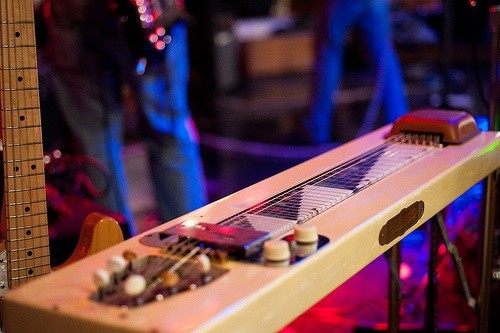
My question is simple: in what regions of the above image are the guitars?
[0,1,126,295]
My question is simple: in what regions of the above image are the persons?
[303,0,411,146]
[48,0,204,235]
